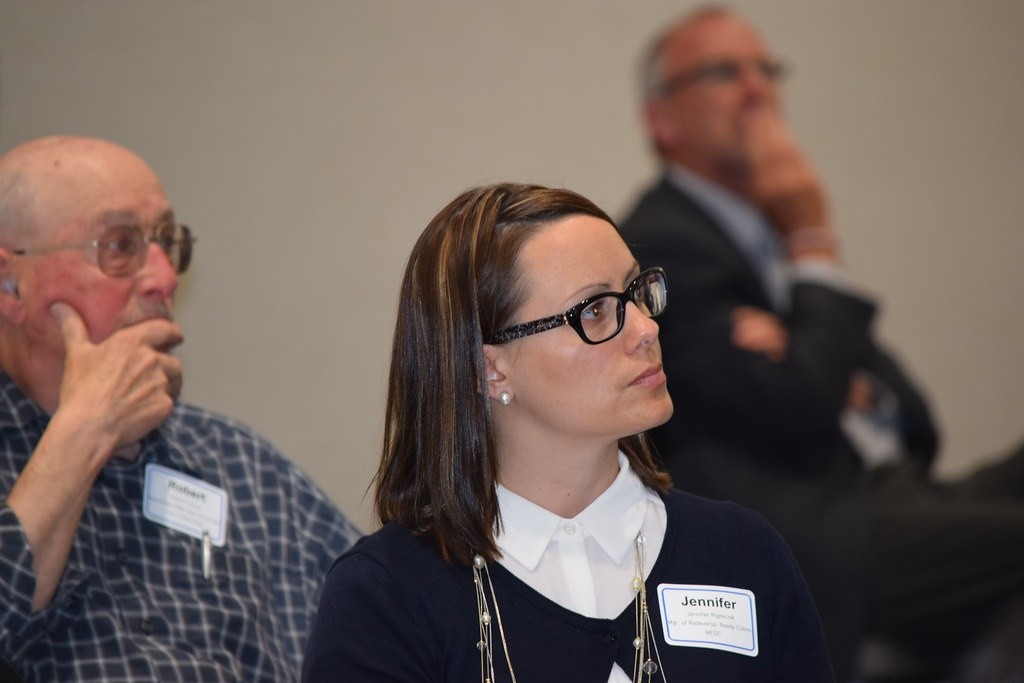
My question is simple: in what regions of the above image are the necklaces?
[472,533,666,683]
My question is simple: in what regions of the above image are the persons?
[616,12,1023,683]
[300,182,835,683]
[0,135,363,683]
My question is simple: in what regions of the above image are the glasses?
[484,266,668,345]
[6,222,197,277]
[659,56,788,97]
